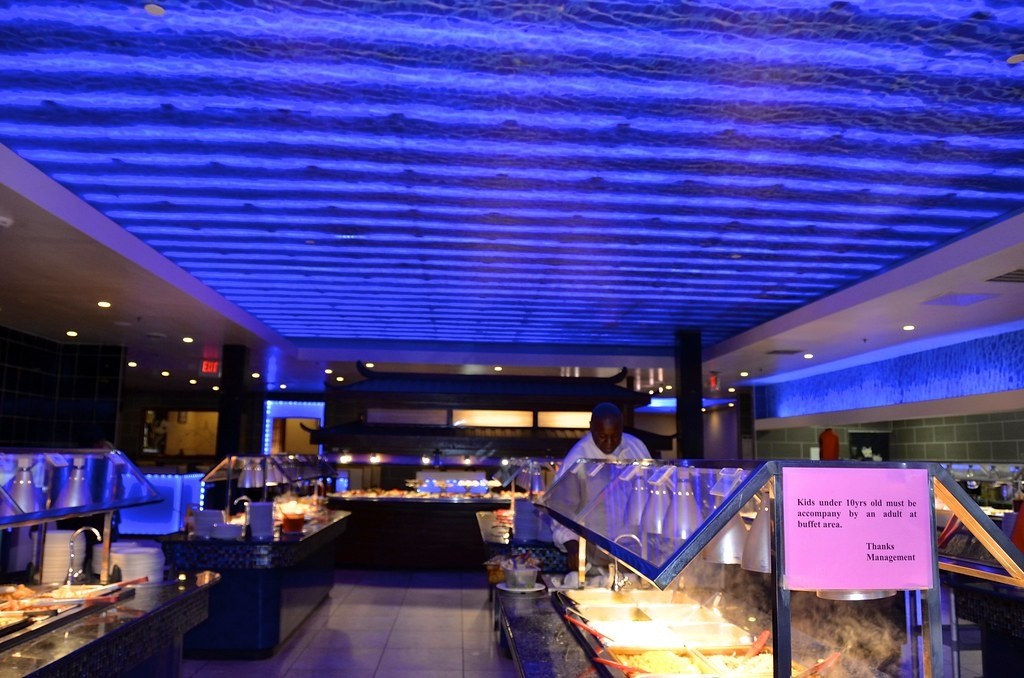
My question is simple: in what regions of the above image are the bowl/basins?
[503,567,538,588]
[283,512,306,533]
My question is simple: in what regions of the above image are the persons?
[547,402,671,585]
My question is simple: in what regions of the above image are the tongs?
[31,596,120,607]
[77,575,148,595]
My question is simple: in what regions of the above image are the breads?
[0,584,34,601]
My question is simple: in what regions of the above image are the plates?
[496,582,547,592]
[194,510,242,540]
[30,529,165,584]
[249,501,275,540]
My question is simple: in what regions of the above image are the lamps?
[51,458,92,509]
[0,458,35,515]
[517,466,546,492]
[967,464,1024,489]
[623,470,898,601]
[238,462,323,488]
